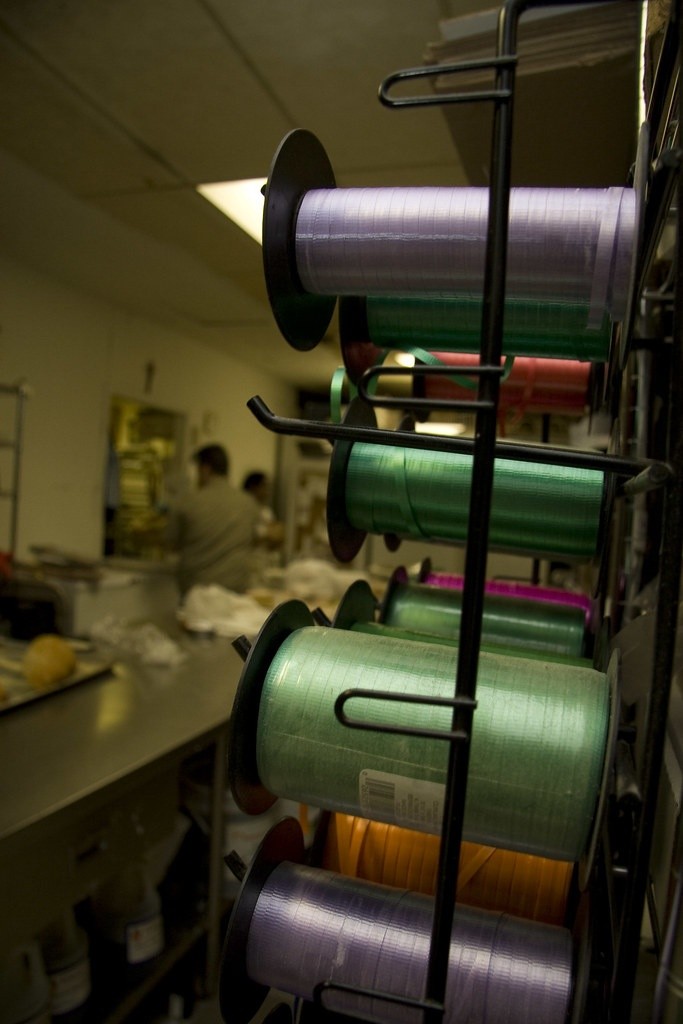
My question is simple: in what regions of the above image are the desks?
[0,611,298,1024]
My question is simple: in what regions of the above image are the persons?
[163,445,276,642]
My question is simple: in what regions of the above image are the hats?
[195,446,227,474]
